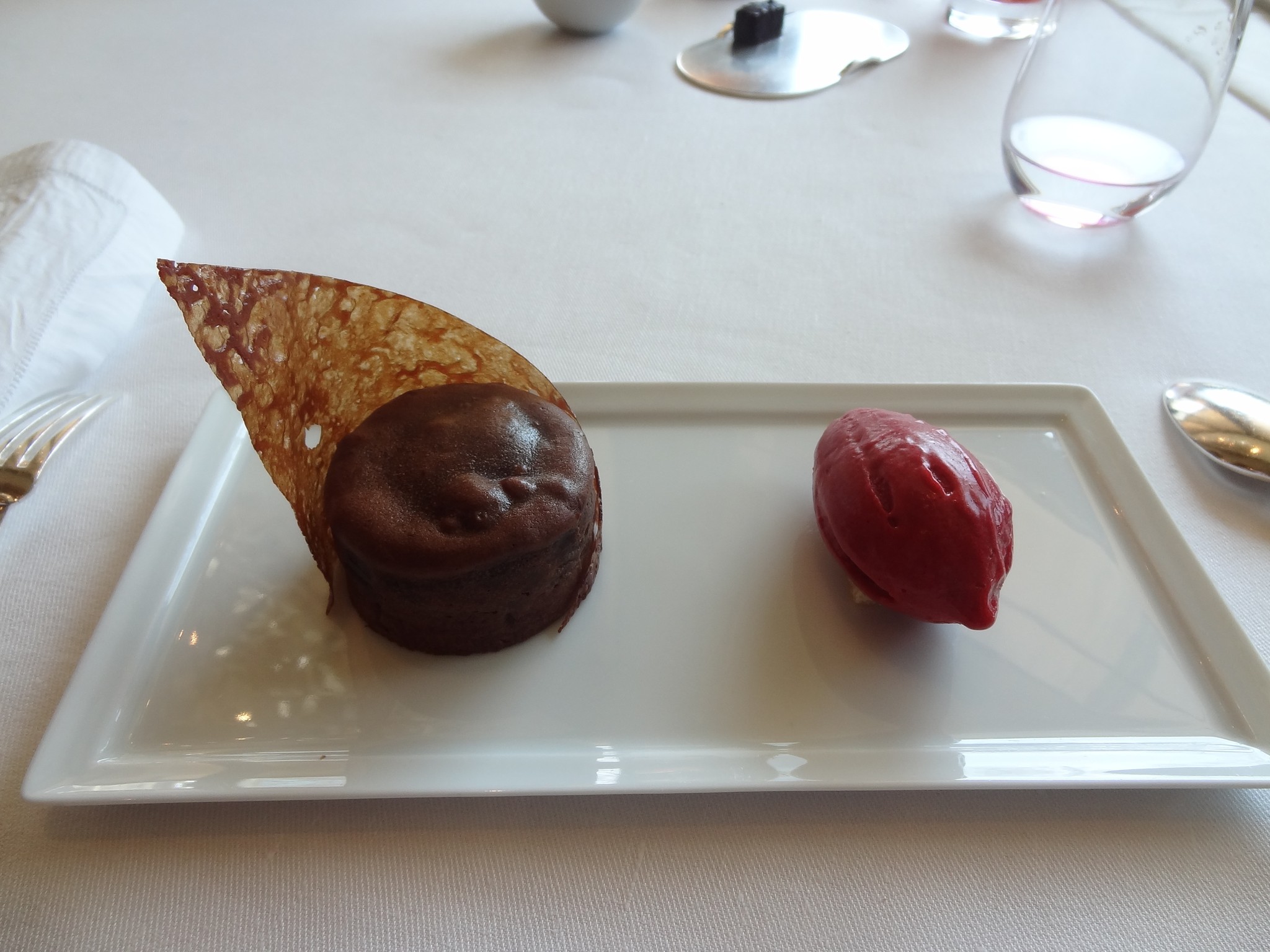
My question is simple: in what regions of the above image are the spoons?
[1163,379,1270,481]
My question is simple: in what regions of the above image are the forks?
[0,387,107,522]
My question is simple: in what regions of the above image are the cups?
[1003,0,1253,229]
[948,0,1062,40]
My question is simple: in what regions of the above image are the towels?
[0,134,187,429]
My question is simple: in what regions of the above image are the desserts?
[155,258,605,658]
[813,406,1013,630]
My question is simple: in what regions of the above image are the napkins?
[0,140,185,417]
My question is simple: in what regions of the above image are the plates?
[20,380,1270,808]
